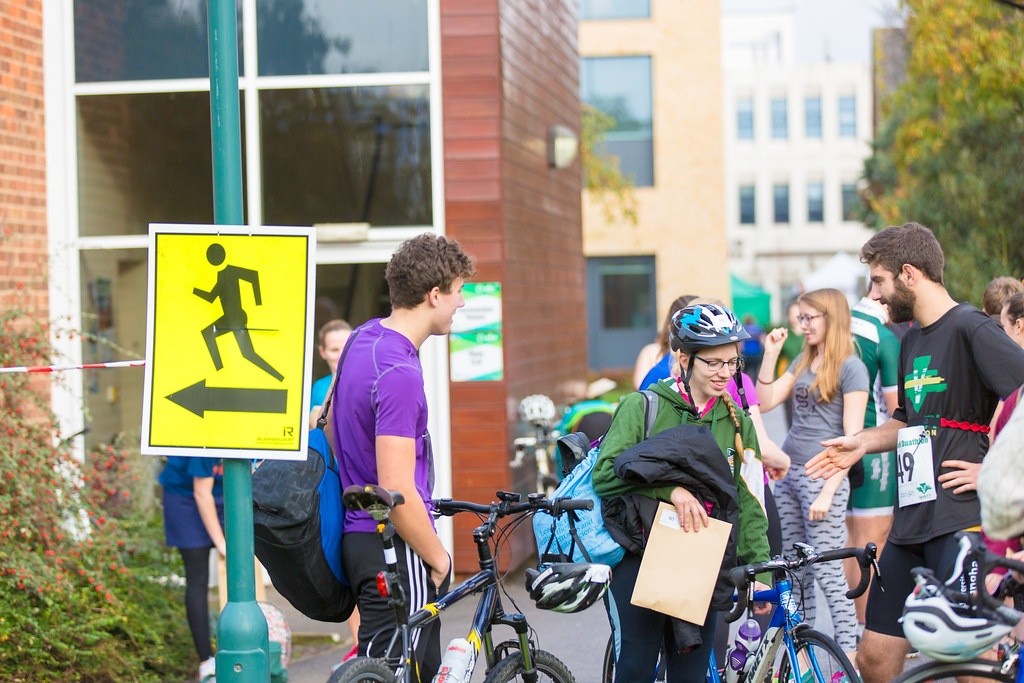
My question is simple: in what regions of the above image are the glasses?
[695,355,741,370]
[797,314,826,326]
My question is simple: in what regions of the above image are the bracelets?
[757,377,773,385]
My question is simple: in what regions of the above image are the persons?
[805,222,1024,683]
[634,295,791,582]
[755,289,870,676]
[554,399,615,478]
[981,275,1024,610]
[318,234,473,683]
[742,316,762,370]
[308,319,360,674]
[593,303,774,683]
[843,279,902,626]
[769,292,806,432]
[159,456,227,683]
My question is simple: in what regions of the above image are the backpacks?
[532,392,659,569]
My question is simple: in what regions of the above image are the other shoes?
[200,657,216,683]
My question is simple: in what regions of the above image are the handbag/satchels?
[252,429,355,622]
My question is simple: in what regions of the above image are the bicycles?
[602,532,1023,683]
[327,483,595,683]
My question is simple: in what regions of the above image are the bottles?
[431,637,473,683]
[724,619,762,683]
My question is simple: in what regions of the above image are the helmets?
[519,395,554,422]
[525,562,612,613]
[670,303,751,353]
[898,566,1016,663]
